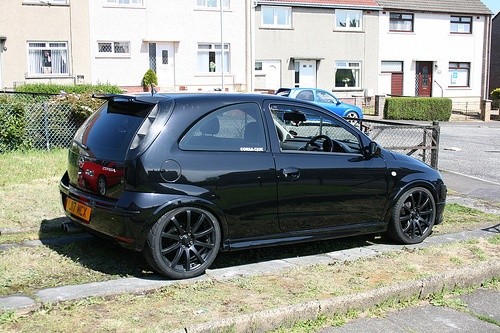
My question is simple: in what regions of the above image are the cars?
[275,88,362,128]
[62,91,448,280]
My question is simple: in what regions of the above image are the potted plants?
[209,62,215,72]
[342,78,350,87]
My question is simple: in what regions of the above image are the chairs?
[195,116,223,147]
[244,124,267,148]
